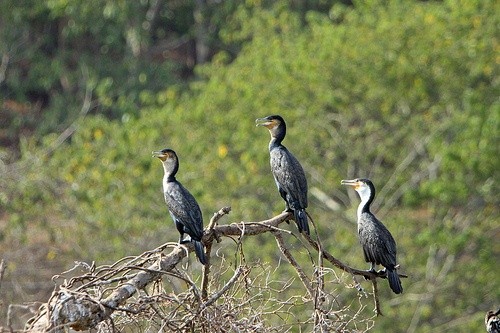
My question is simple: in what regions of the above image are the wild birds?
[340,178,403,295]
[151,148,205,266]
[255,115,311,236]
